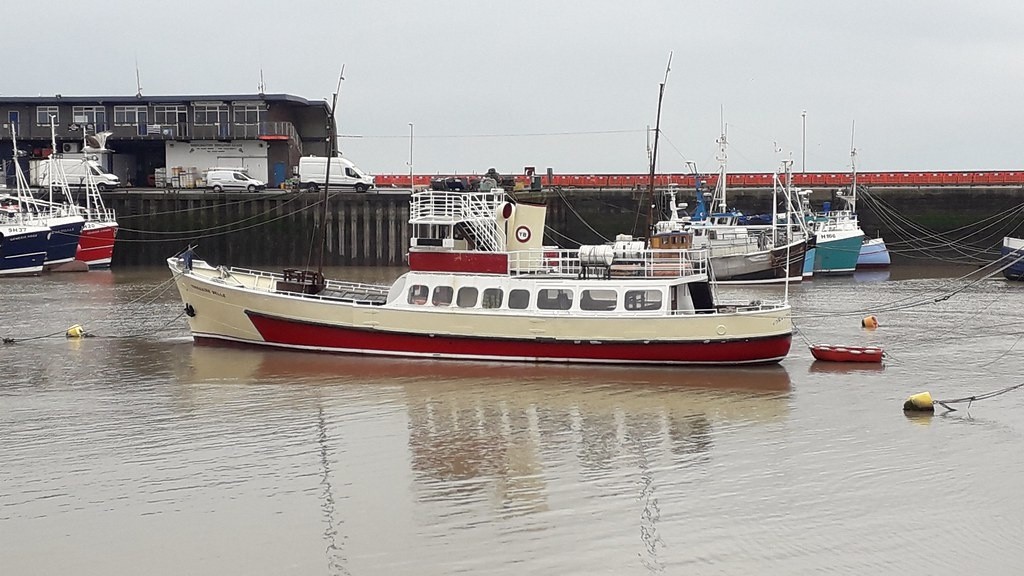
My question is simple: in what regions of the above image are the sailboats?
[577,105,891,285]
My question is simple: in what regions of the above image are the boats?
[167,191,797,366]
[810,343,885,362]
[1,114,119,276]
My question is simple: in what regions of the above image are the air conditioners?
[63,143,77,153]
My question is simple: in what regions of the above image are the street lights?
[407,123,413,189]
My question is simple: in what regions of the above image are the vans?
[207,168,265,193]
[297,157,376,193]
[39,159,121,191]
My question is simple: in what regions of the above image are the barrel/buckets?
[579,245,613,266]
[613,240,646,258]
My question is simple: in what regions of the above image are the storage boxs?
[155,167,207,188]
